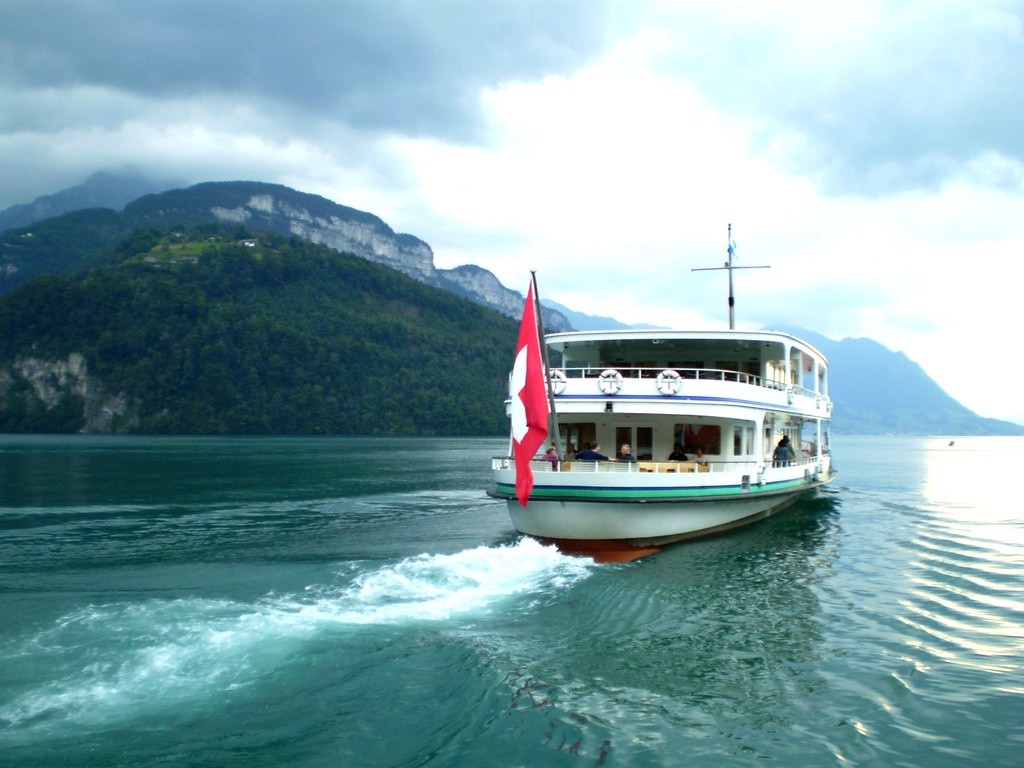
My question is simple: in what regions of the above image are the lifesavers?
[597,369,624,395]
[544,370,568,395]
[656,369,683,397]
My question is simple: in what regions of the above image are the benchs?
[508,457,709,473]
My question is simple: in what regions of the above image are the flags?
[513,281,549,507]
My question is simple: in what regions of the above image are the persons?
[543,443,559,472]
[616,444,637,472]
[568,444,576,455]
[583,443,590,450]
[773,435,796,467]
[574,442,611,472]
[667,446,693,472]
[694,449,706,466]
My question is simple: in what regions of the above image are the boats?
[485,222,838,566]
